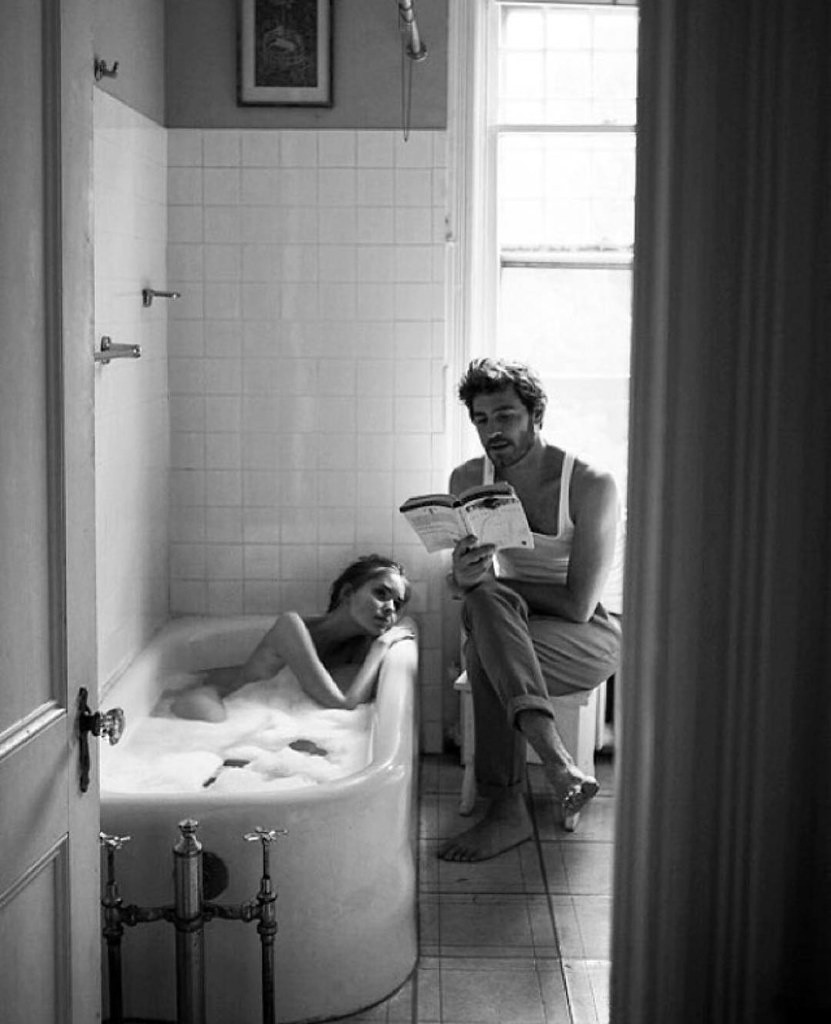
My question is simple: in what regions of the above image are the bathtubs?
[99,609,423,1024]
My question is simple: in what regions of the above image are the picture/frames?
[237,0,333,107]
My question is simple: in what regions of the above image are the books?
[397,479,535,554]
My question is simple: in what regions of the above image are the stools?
[451,665,608,834]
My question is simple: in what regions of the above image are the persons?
[169,554,416,724]
[436,357,625,863]
